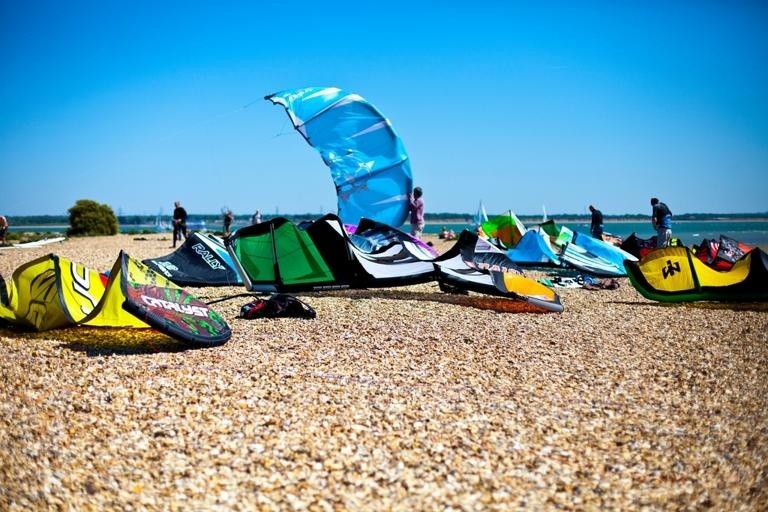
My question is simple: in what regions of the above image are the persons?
[408,187,425,241]
[251,209,262,225]
[224,208,233,234]
[169,201,188,247]
[0,216,8,244]
[650,198,673,249]
[448,229,456,239]
[441,226,448,239]
[589,205,603,241]
